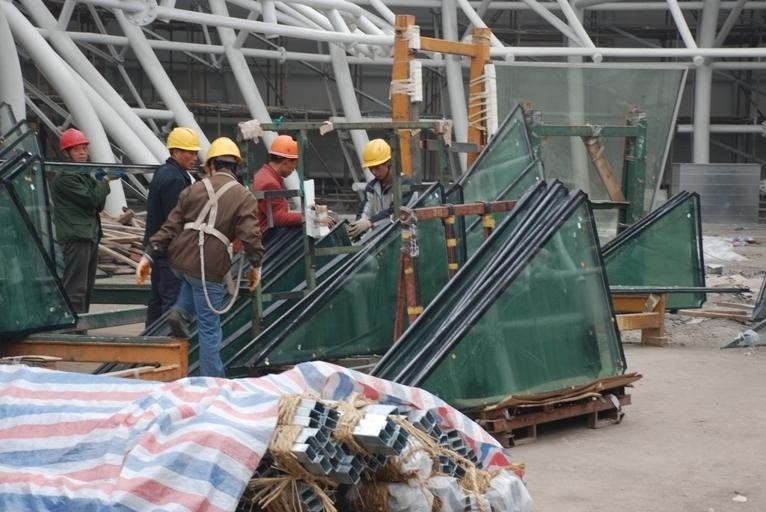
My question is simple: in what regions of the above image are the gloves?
[136,255,150,285]
[89,169,109,185]
[348,217,368,241]
[249,265,262,292]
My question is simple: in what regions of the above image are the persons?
[232,135,336,257]
[136,137,264,380]
[348,139,419,243]
[51,128,125,336]
[143,127,203,329]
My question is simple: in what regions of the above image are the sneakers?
[168,306,191,338]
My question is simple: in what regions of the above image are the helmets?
[60,128,89,151]
[361,138,392,168]
[269,134,299,159]
[206,137,242,166]
[166,127,201,151]
